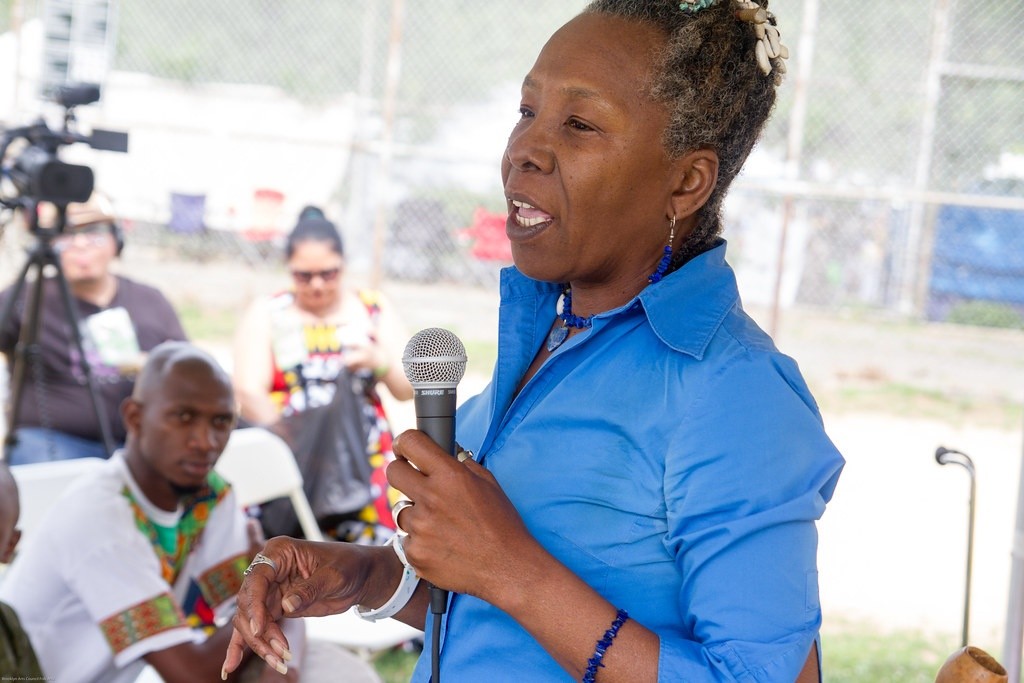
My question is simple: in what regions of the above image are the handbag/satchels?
[232,364,381,543]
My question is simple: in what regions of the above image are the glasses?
[292,269,340,284]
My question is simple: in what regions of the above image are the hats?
[36,188,112,230]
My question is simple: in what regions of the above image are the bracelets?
[582,609,630,683]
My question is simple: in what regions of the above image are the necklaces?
[546,281,597,355]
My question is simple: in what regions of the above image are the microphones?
[401,327,468,614]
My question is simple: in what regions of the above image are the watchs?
[354,531,422,624]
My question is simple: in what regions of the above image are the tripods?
[0,238,118,465]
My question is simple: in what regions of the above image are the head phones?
[25,220,124,267]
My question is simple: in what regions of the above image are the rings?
[456,450,474,463]
[392,502,414,531]
[245,552,279,577]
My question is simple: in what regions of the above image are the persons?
[235,200,419,547]
[0,337,384,683]
[222,0,846,683]
[0,200,194,463]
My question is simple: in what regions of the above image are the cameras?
[1,83,128,203]
